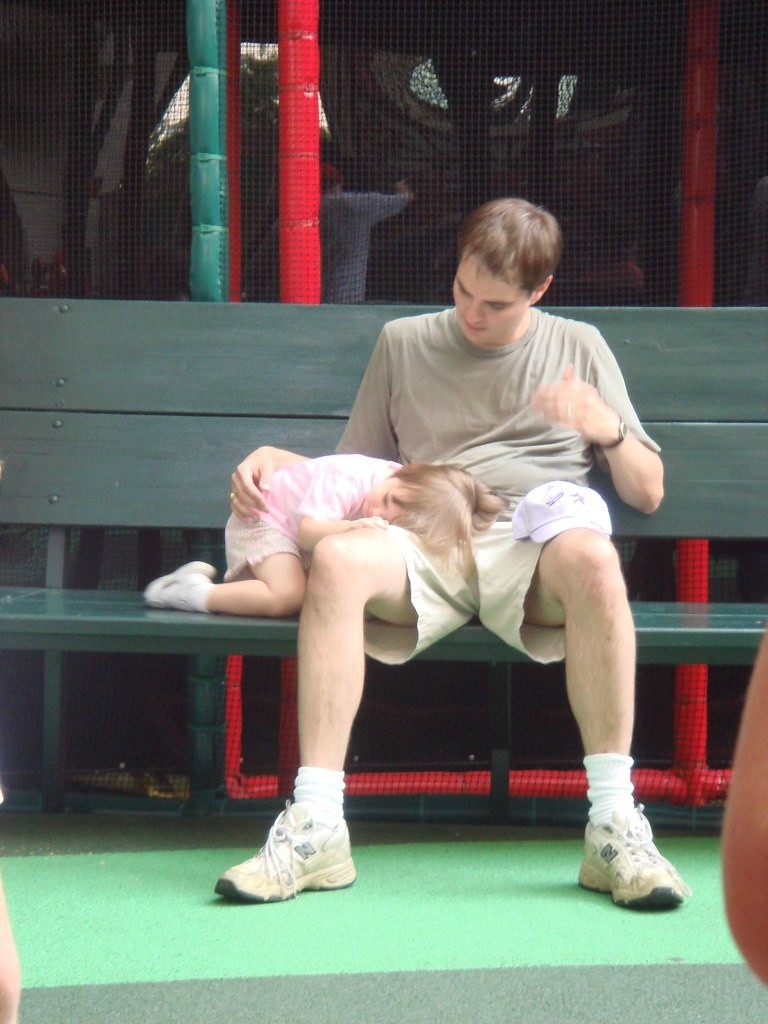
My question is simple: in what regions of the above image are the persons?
[142,454,510,618]
[320,166,413,303]
[215,197,685,910]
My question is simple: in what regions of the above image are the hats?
[508,481,615,545]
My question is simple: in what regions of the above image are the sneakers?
[213,799,358,903]
[578,803,694,908]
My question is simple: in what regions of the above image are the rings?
[229,491,236,502]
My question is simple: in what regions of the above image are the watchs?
[601,414,627,450]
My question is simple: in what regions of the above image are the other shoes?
[142,559,216,608]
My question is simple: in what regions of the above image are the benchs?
[0,296,768,664]
